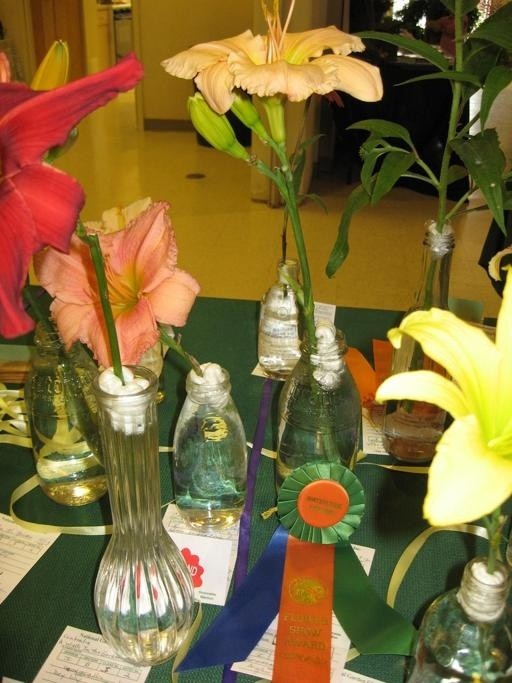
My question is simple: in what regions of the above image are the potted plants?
[325,0,512,461]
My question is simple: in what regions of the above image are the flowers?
[160,2,384,464]
[29,196,220,381]
[282,88,343,299]
[1,34,147,382]
[0,53,142,465]
[373,262,512,573]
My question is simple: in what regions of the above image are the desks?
[2,295,512,682]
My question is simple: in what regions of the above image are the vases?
[24,322,106,507]
[257,257,303,375]
[382,222,457,466]
[405,558,511,683]
[87,367,193,666]
[174,363,248,531]
[277,329,360,502]
